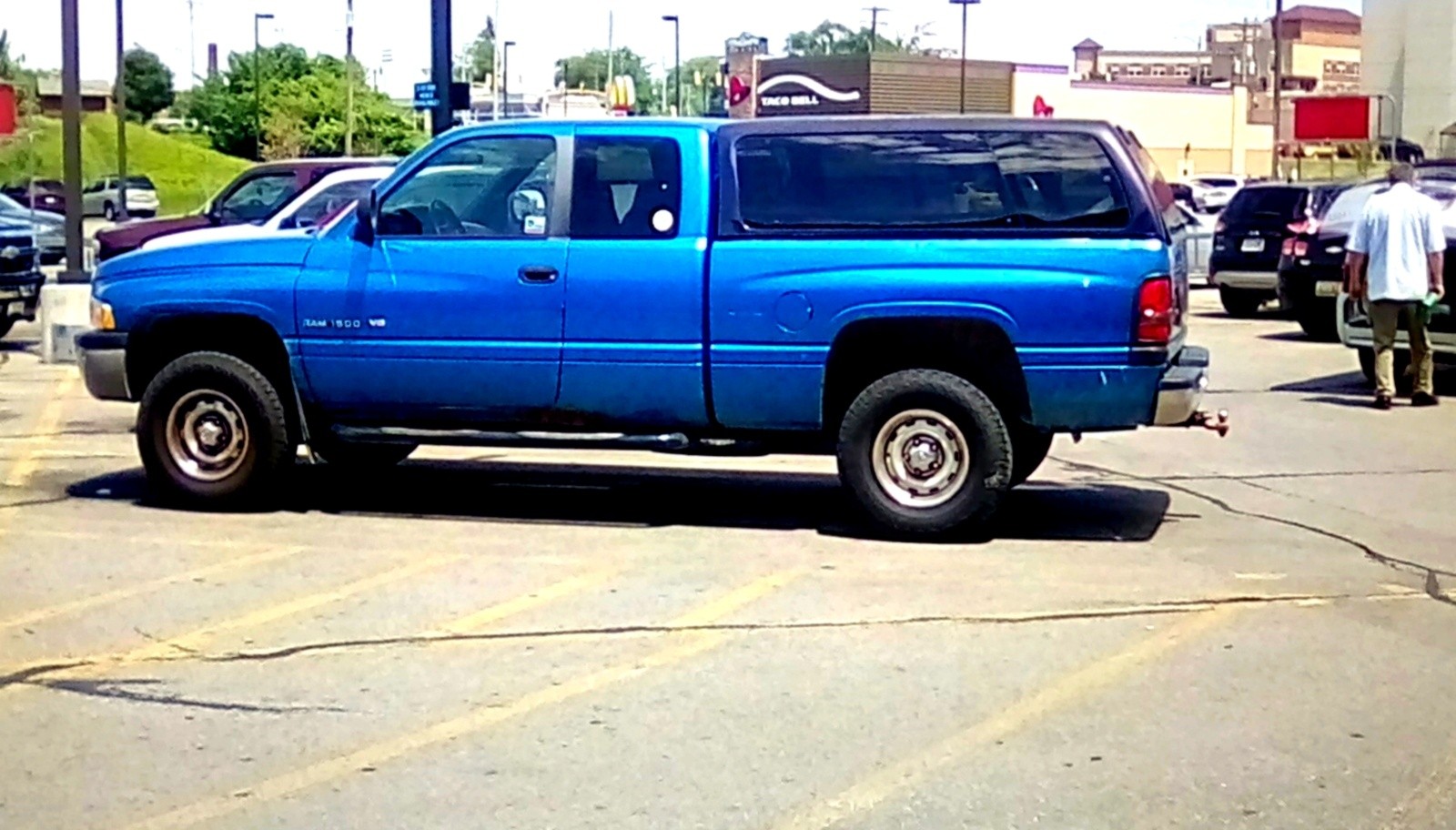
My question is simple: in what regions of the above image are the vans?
[1209,177,1341,320]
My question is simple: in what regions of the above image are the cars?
[140,166,508,251]
[1167,175,1241,289]
[0,178,67,214]
[90,155,401,262]
[82,172,160,222]
[0,194,68,266]
[1277,159,1456,398]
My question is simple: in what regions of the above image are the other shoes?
[1412,392,1437,405]
[1375,395,1390,407]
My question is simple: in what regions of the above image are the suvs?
[0,218,45,339]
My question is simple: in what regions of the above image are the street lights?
[863,9,890,51]
[664,16,679,115]
[503,42,517,118]
[948,0,979,113]
[255,12,274,160]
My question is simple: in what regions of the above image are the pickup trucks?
[71,114,1233,543]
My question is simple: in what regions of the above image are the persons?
[1346,163,1446,407]
